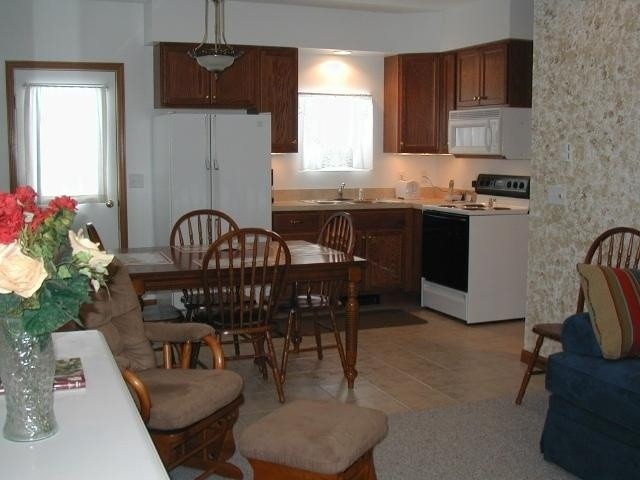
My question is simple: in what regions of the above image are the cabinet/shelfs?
[383,52,445,154]
[258,46,299,154]
[152,43,258,111]
[452,37,533,111]
[272,212,324,296]
[324,210,408,298]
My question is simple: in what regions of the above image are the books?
[0,357,87,394]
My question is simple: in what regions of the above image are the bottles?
[471,180,477,203]
[448,178,453,193]
[359,189,364,200]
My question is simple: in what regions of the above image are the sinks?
[316,201,355,205]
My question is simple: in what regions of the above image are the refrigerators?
[151,110,273,246]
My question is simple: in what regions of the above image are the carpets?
[267,308,429,338]
[167,385,588,480]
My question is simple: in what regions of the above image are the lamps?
[187,0,245,82]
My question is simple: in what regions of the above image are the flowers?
[0,185,116,356]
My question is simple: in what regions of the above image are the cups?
[464,192,472,202]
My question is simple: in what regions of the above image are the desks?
[1,328,172,480]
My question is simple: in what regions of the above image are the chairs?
[514,226,640,403]
[189,227,292,404]
[168,207,246,369]
[84,222,187,369]
[57,253,245,480]
[268,212,359,386]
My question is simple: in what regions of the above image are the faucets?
[337,183,345,199]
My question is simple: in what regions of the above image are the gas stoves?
[424,202,528,214]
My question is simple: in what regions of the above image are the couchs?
[536,312,640,479]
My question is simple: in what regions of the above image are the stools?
[237,400,391,480]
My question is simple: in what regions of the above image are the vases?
[1,308,60,443]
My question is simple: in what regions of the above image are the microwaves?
[448,108,533,162]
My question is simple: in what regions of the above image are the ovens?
[422,209,468,323]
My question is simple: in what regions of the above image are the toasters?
[395,180,423,199]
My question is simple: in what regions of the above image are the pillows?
[574,263,640,362]
